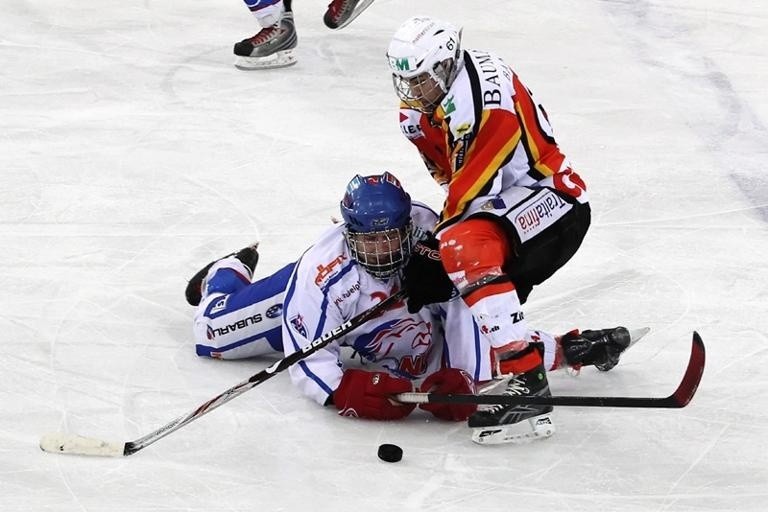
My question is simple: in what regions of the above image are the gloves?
[419,365,477,424]
[400,231,454,315]
[329,362,418,422]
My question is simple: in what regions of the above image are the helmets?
[382,14,465,114]
[338,170,413,282]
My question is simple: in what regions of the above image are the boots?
[182,245,259,307]
[232,0,298,59]
[562,322,631,376]
[468,342,556,430]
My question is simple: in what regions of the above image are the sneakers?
[323,0,361,30]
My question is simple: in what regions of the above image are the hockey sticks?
[41,290,402,457]
[396,332,707,407]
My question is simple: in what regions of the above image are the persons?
[385,12,594,429]
[230,1,361,61]
[182,172,631,420]
